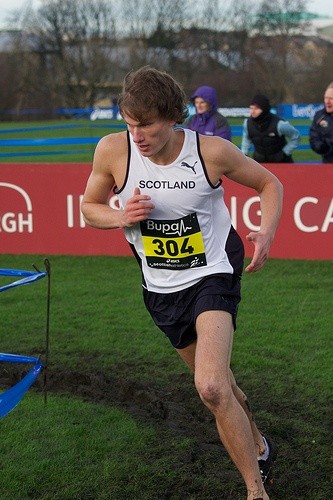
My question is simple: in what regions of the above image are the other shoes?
[256,435,277,487]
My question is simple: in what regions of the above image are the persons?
[309,82,333,163]
[80,66,284,500]
[241,94,302,163]
[185,86,232,143]
